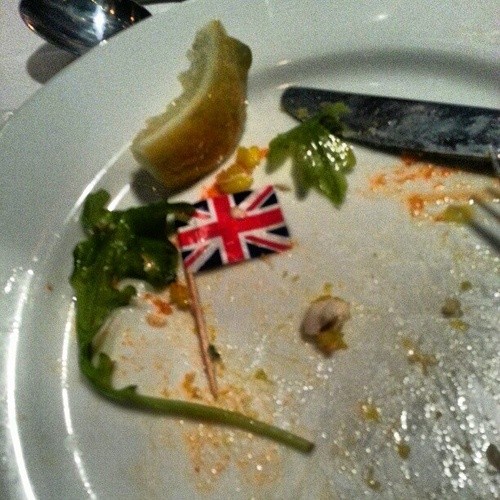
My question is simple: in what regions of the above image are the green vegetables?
[68,187,316,454]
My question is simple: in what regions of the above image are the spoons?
[17,0,153,58]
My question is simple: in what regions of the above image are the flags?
[175,186,294,276]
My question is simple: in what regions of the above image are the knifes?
[280,85,499,163]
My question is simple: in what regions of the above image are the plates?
[1,0,498,500]
